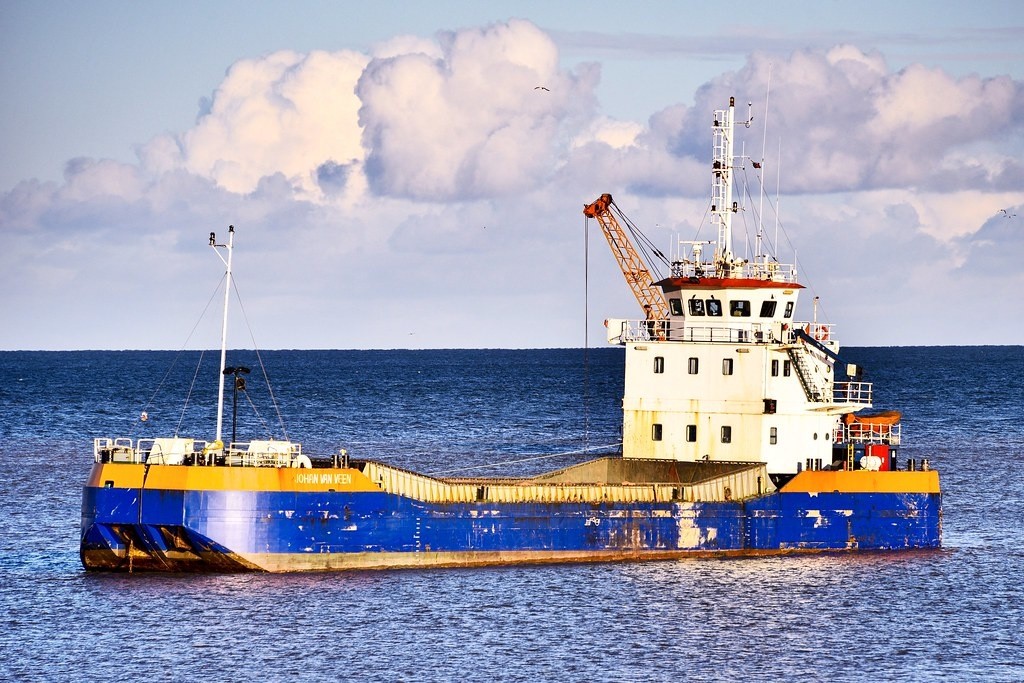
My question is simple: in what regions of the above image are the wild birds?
[534,87,551,92]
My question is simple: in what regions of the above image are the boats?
[79,91,945,574]
[845,410,902,433]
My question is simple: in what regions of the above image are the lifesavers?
[814,327,828,340]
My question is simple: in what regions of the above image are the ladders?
[848,444,854,471]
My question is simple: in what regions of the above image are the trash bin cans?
[921,459,929,471]
[908,458,916,471]
[806,458,813,471]
[815,459,822,471]
